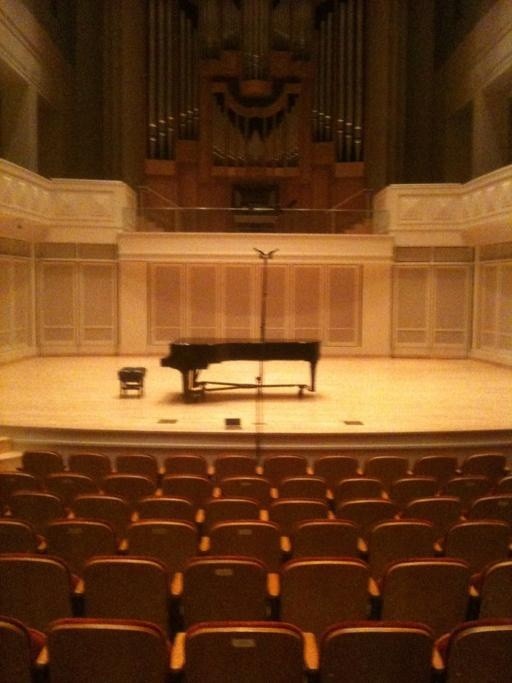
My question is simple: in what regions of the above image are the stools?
[118,368,146,399]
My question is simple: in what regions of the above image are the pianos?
[161,337,320,401]
